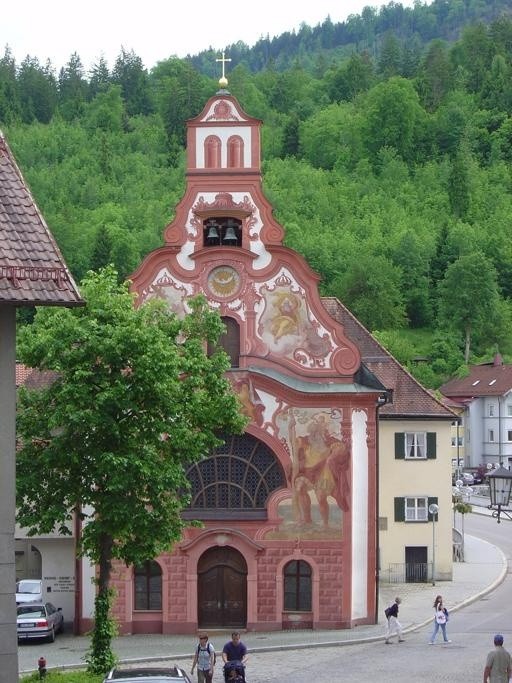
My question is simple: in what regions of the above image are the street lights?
[428,503,439,587]
[451,460,500,562]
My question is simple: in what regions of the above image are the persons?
[483,634,512,682]
[222,631,249,664]
[291,412,347,534]
[429,595,453,644]
[384,596,406,644]
[228,668,243,683]
[190,631,216,682]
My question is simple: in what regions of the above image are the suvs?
[101,663,192,683]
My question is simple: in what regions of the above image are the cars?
[15,600,66,643]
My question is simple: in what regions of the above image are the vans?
[15,578,43,607]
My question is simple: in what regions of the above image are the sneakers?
[445,640,451,643]
[385,641,392,644]
[428,642,436,645]
[399,640,404,642]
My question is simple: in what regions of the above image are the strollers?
[223,658,247,683]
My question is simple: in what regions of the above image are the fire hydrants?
[37,656,46,681]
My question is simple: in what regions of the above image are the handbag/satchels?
[385,608,391,618]
[435,611,446,624]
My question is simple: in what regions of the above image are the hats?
[495,635,503,641]
